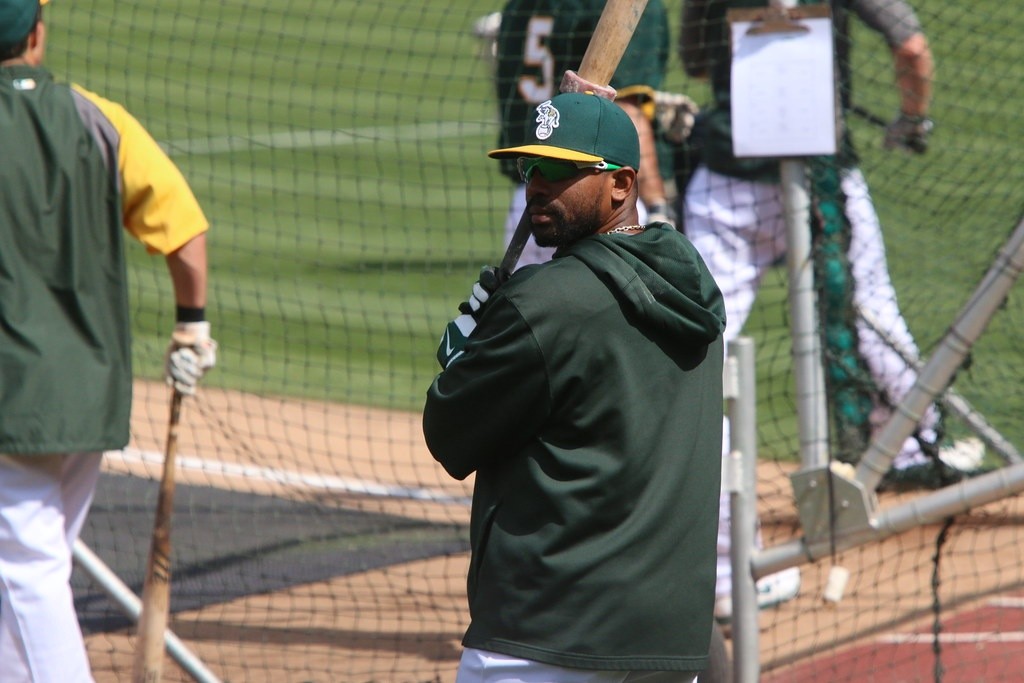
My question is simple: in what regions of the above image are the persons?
[420,95,726,683]
[0,0,216,683]
[491,0,673,272]
[675,1,986,623]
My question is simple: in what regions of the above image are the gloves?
[650,90,698,144]
[436,316,477,371]
[164,305,216,394]
[885,113,934,151]
[457,266,511,321]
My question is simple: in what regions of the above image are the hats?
[486,90,640,174]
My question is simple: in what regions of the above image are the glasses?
[516,151,622,184]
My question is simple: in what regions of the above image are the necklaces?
[607,223,648,235]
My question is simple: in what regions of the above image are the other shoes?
[893,439,988,474]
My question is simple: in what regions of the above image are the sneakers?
[713,565,804,623]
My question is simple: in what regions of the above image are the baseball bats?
[490,0,650,276]
[133,332,198,682]
